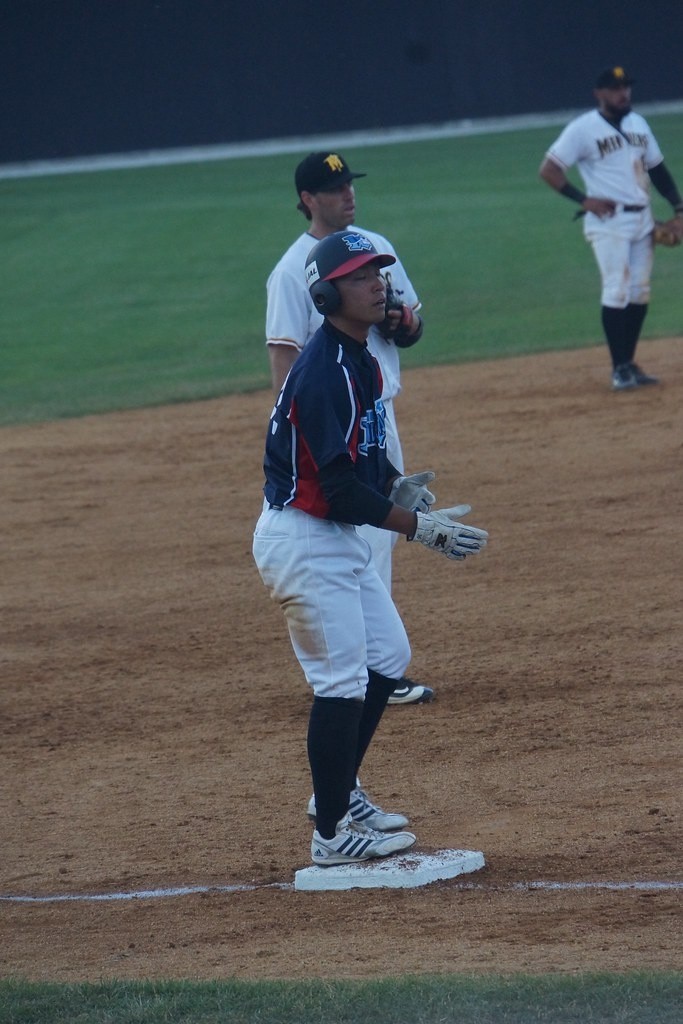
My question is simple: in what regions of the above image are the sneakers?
[386,677,434,704]
[612,361,660,389]
[305,789,416,865]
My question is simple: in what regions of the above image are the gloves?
[387,470,437,514]
[407,504,489,561]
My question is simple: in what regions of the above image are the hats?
[295,152,366,200]
[305,231,397,292]
[594,67,636,88]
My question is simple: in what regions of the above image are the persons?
[541,67,682,390]
[253,230,488,865]
[266,151,433,705]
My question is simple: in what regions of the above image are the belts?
[613,204,645,212]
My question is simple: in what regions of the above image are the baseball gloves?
[654,217,683,247]
